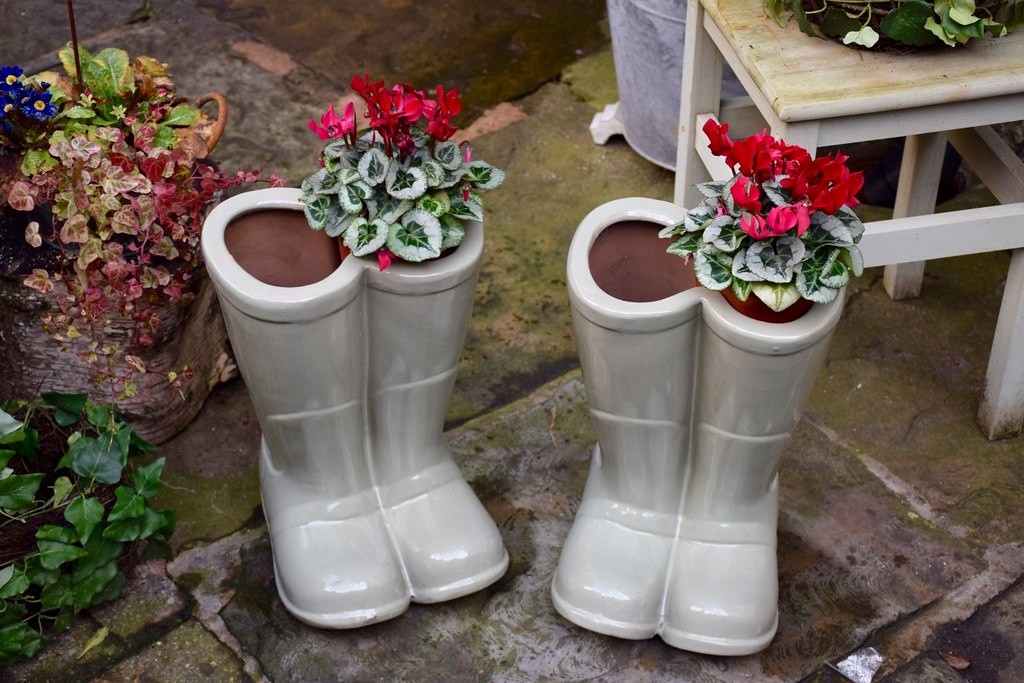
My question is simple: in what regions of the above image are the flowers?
[0,65,58,143]
[657,118,864,312]
[298,73,507,272]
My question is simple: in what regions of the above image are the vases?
[551,197,847,655]
[0,39,287,443]
[201,187,509,629]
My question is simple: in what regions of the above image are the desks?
[673,0,1024,440]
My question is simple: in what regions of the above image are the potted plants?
[0,391,176,666]
[762,0,1007,52]
